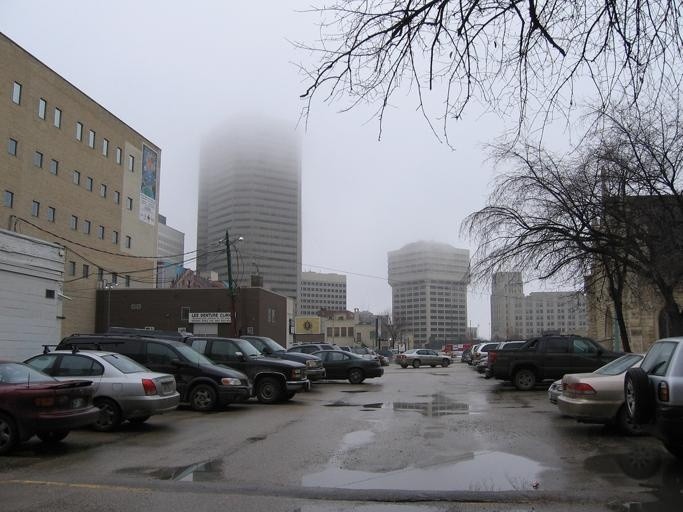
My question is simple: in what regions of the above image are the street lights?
[227,237,244,337]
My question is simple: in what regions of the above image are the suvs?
[57,326,327,413]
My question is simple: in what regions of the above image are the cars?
[547,337,683,435]
[23,342,181,432]
[462,336,623,391]
[395,349,452,368]
[1,360,100,454]
[287,342,389,384]
